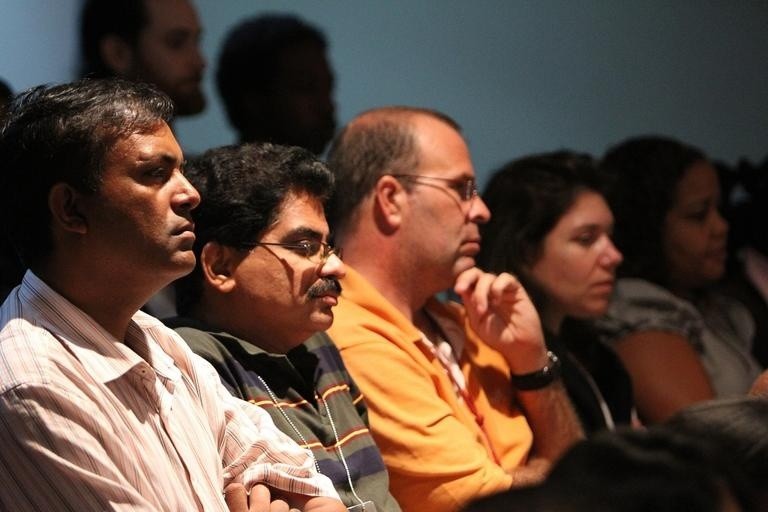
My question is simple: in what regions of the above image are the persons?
[480,149,649,433]
[210,11,339,158]
[324,106,585,511]
[159,143,402,510]
[597,132,768,428]
[1,77,348,510]
[77,1,206,118]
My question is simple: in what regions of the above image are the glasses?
[255,239,345,265]
[383,172,478,202]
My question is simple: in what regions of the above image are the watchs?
[509,352,563,391]
